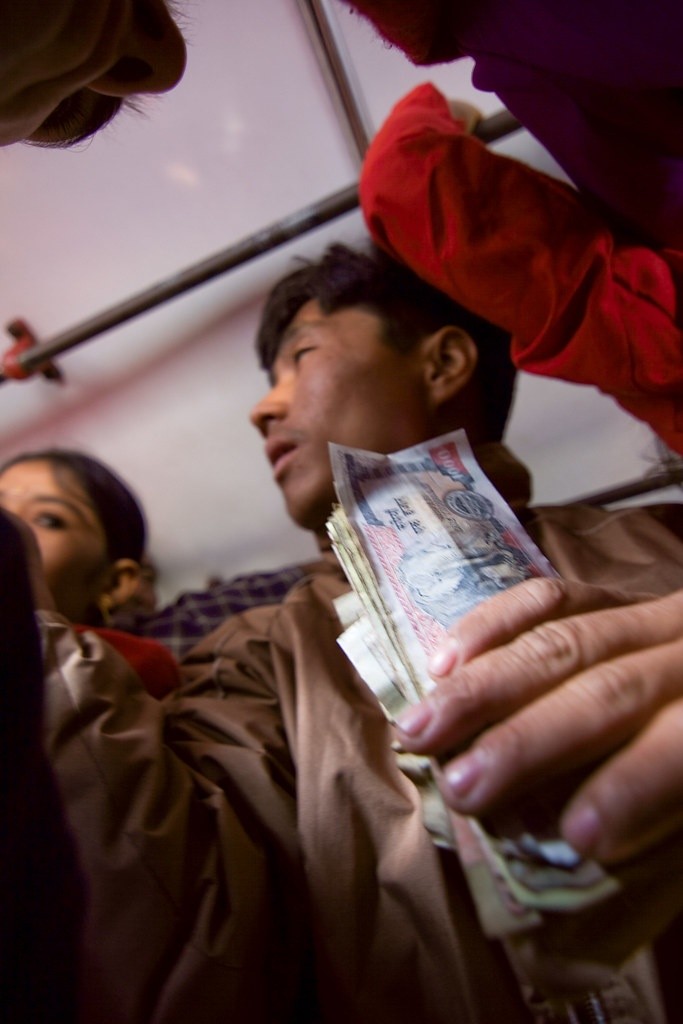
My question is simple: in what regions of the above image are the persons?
[0,0,683,1024]
